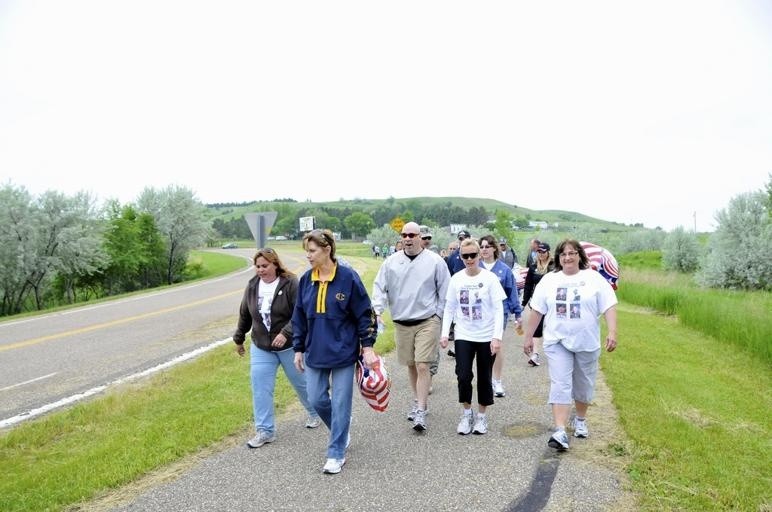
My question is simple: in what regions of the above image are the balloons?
[578,241,619,292]
[512,267,530,291]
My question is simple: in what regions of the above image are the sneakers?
[493,382,505,397]
[247,432,274,448]
[447,348,455,356]
[574,420,588,438]
[548,431,570,450]
[528,357,540,366]
[472,413,488,434]
[306,416,320,427]
[408,374,434,431]
[322,416,352,474]
[457,407,474,434]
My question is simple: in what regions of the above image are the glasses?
[462,253,477,259]
[422,236,432,240]
[401,233,419,238]
[480,245,493,249]
[560,252,579,257]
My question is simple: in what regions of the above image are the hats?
[420,226,433,238]
[537,243,550,252]
[458,230,471,239]
[500,239,506,245]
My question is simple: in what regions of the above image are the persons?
[372,225,541,267]
[371,221,451,429]
[232,247,323,449]
[521,243,555,365]
[476,235,522,398]
[439,236,508,435]
[523,238,619,449]
[289,229,377,473]
